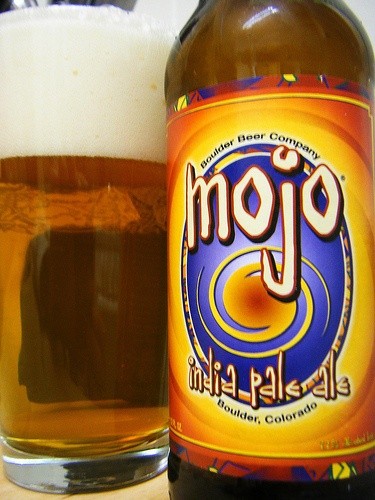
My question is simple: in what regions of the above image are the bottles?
[162,0,375,500]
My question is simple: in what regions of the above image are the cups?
[1,6,176,495]
